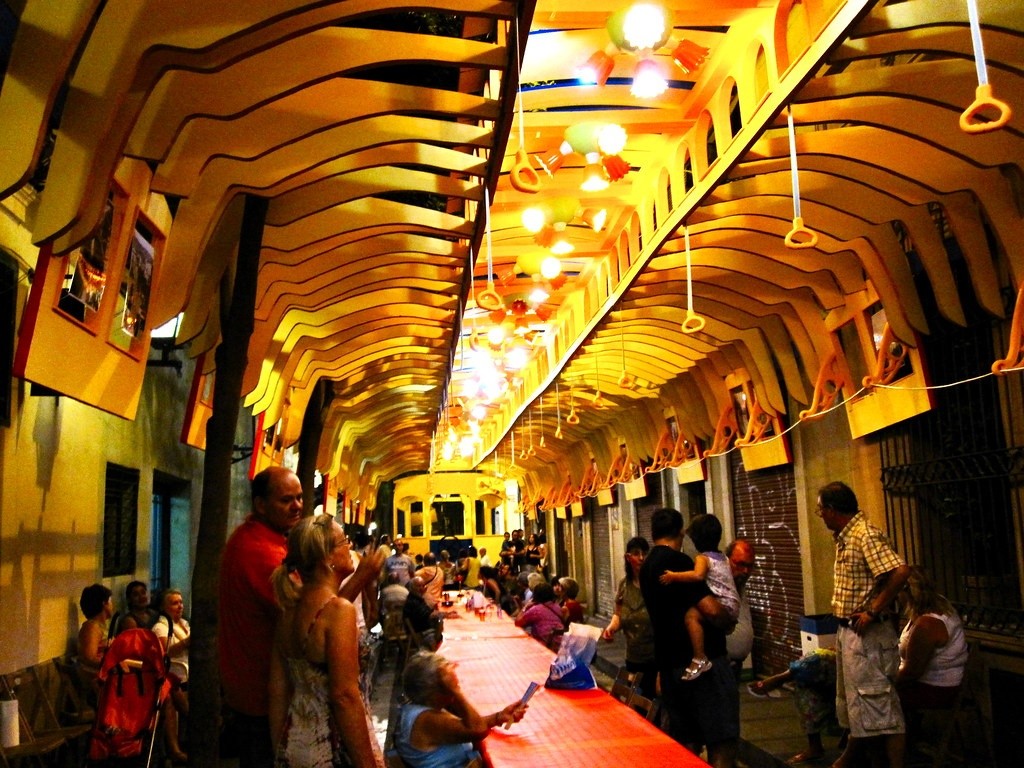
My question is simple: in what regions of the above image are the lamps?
[534,119,631,193]
[440,250,569,462]
[521,196,607,255]
[578,2,712,99]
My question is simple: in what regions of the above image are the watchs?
[865,607,879,618]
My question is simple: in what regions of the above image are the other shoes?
[786,747,823,764]
[747,679,782,698]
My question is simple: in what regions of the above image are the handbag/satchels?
[542,623,604,691]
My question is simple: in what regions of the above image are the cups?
[445,592,449,603]
[479,607,485,622]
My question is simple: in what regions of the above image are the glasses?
[813,505,828,517]
[333,534,350,548]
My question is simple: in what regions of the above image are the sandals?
[680,658,713,681]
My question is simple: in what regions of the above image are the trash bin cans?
[800,613,840,655]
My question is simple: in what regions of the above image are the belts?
[837,617,857,628]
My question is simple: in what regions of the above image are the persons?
[76,581,190,765]
[749,565,969,768]
[394,651,529,768]
[814,482,911,768]
[269,514,379,768]
[218,467,385,768]
[602,537,662,729]
[638,508,755,768]
[344,528,587,664]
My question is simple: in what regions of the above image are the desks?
[437,589,715,768]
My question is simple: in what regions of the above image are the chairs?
[629,687,662,723]
[908,638,979,768]
[0,634,101,768]
[383,599,422,685]
[610,666,642,705]
[384,707,482,768]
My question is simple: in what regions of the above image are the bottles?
[497,607,502,618]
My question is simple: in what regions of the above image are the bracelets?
[494,711,504,727]
